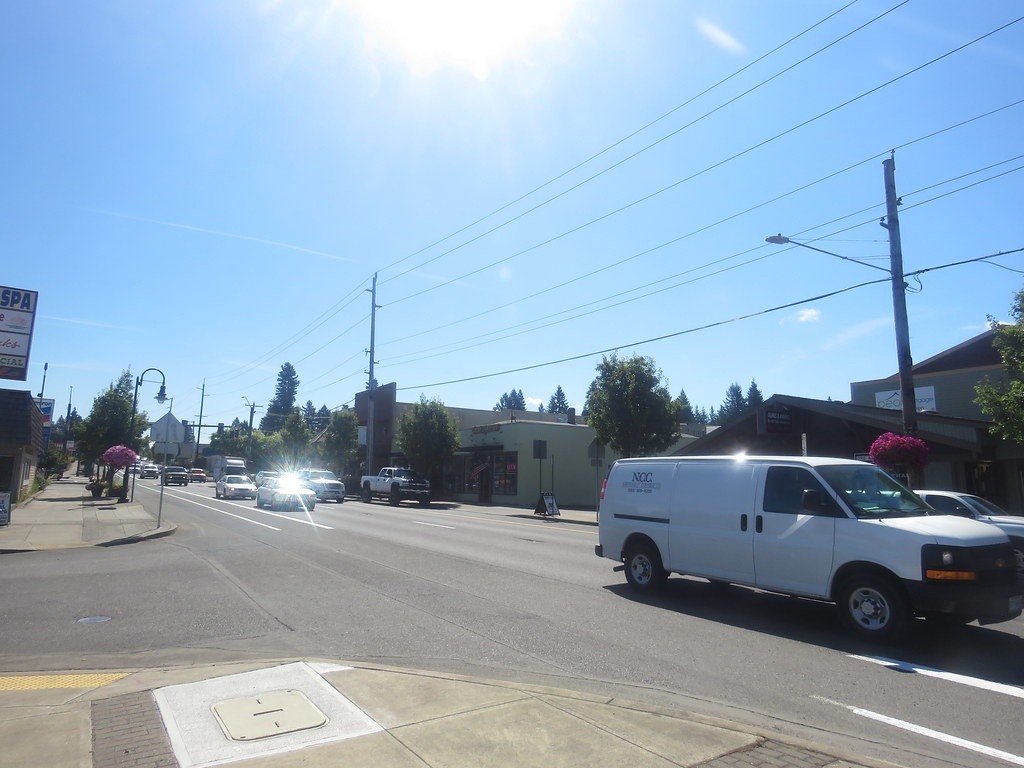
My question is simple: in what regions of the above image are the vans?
[595,456,1024,644]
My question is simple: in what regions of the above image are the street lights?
[241,397,256,452]
[118,368,170,503]
[765,233,924,490]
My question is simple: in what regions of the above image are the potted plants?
[104,477,129,496]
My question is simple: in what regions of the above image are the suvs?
[255,471,280,489]
[140,465,159,479]
[298,470,345,503]
[161,466,188,486]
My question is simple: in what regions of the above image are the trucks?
[214,456,246,482]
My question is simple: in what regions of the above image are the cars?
[129,460,140,473]
[188,468,206,483]
[216,475,258,500]
[257,480,316,511]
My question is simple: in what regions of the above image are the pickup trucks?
[361,467,431,507]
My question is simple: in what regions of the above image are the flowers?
[868,433,930,475]
[103,445,138,469]
[85,482,107,490]
[92,489,103,497]
